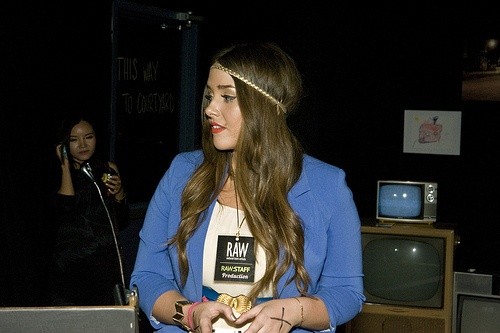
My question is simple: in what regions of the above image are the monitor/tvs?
[360,226,454,310]
[377,180,438,223]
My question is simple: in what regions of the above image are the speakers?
[343,302,451,333]
[0,308,140,333]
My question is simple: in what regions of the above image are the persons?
[130,40,366,333]
[46,113,129,305]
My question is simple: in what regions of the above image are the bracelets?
[187,295,208,332]
[292,296,304,327]
[171,300,194,332]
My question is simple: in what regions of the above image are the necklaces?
[229,166,247,242]
